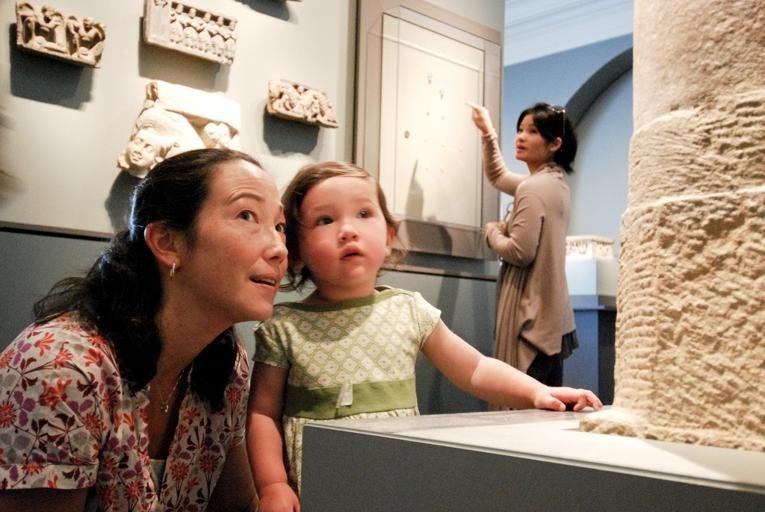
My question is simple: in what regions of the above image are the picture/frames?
[351,0,505,261]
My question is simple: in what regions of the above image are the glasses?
[547,102,567,138]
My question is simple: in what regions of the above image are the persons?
[0,147,293,512]
[242,160,604,512]
[463,100,580,411]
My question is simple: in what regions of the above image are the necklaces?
[150,366,185,413]
